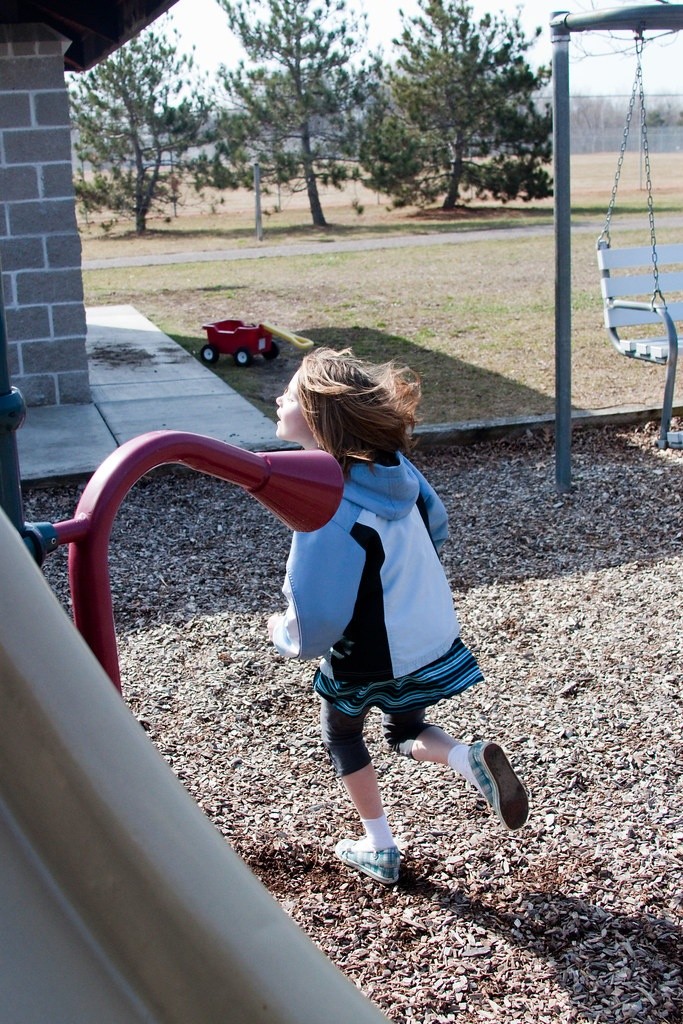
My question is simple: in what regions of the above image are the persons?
[268,347,528,885]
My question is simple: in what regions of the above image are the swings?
[596,34,683,367]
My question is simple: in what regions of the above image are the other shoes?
[467,741,529,831]
[335,839,400,882]
